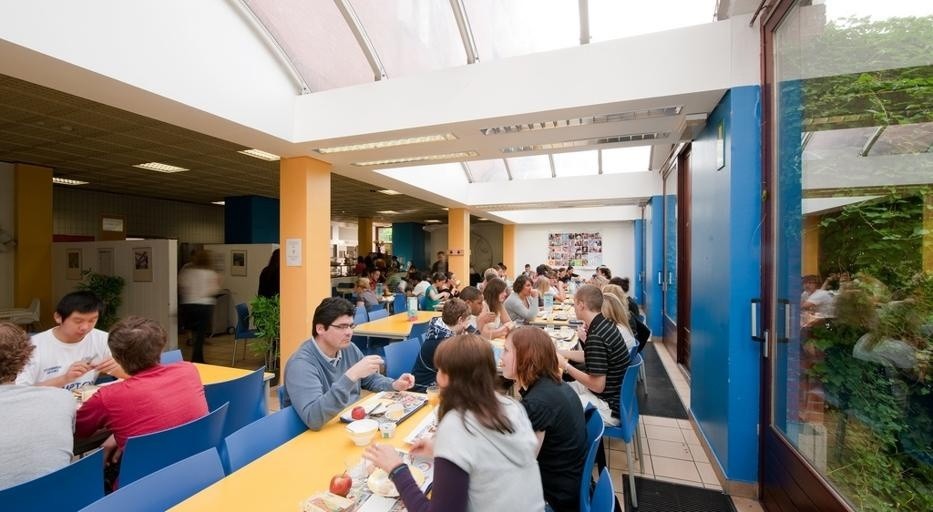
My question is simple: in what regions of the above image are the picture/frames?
[64,246,83,280]
[94,245,117,278]
[228,248,247,278]
[130,246,154,283]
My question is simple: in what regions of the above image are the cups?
[426,386,439,407]
[543,292,574,342]
[384,404,404,423]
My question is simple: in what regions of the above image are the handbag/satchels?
[584,405,623,511]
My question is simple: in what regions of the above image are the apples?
[329,470,352,498]
[352,406,365,420]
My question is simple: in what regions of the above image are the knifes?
[367,403,381,414]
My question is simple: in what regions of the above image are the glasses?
[331,322,356,329]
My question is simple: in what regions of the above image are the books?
[402,410,438,449]
[301,441,433,512]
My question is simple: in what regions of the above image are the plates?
[365,465,424,499]
[363,398,395,414]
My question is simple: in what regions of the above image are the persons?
[258,249,280,297]
[74,316,209,492]
[499,327,587,511]
[361,335,546,512]
[15,292,132,391]
[0,322,75,489]
[283,296,416,430]
[349,249,651,431]
[178,242,221,364]
[798,273,933,422]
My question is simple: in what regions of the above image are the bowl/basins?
[344,418,377,447]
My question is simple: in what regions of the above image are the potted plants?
[249,294,281,388]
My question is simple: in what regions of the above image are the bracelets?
[387,463,410,479]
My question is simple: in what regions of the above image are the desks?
[164,390,440,511]
[75,361,275,425]
[356,310,443,347]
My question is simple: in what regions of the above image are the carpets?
[636,341,688,423]
[620,472,737,511]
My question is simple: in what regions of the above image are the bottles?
[488,313,495,324]
[379,422,396,439]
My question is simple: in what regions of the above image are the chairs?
[118,400,231,486]
[158,350,182,365]
[1,447,106,512]
[354,293,406,358]
[601,339,649,507]
[79,447,226,511]
[383,337,422,379]
[408,320,430,339]
[581,401,618,511]
[203,367,270,437]
[231,302,272,371]
[225,406,311,473]
